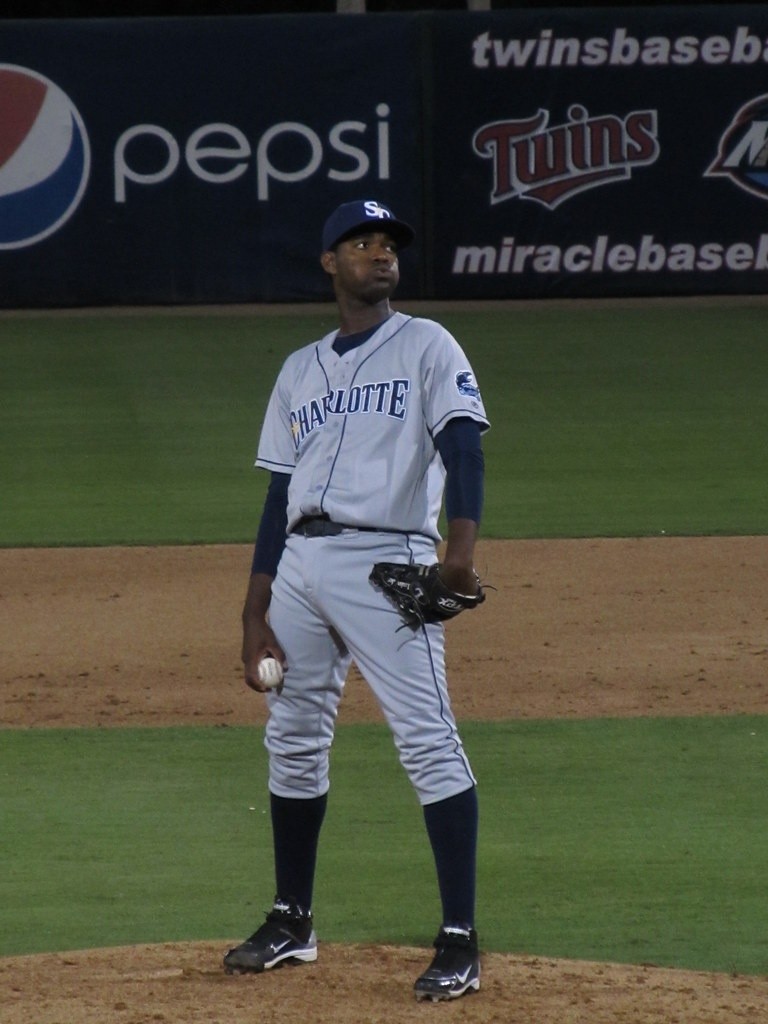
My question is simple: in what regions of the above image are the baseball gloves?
[370,561,500,634]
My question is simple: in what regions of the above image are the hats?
[322,200,417,251]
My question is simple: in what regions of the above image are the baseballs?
[258,658,284,689]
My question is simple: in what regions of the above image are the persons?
[224,200,491,1001]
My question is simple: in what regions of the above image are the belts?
[290,517,417,537]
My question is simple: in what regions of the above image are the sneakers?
[223,900,317,976]
[414,922,480,1003]
